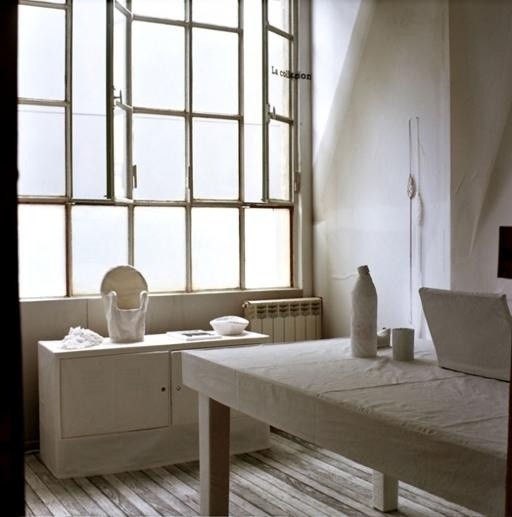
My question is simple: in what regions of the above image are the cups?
[390,327,415,362]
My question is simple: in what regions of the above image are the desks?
[181,336,512,517]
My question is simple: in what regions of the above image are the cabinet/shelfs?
[36,330,272,479]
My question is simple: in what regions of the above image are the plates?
[209,315,249,337]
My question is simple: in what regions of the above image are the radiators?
[243,296,326,339]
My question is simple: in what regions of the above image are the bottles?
[347,264,376,358]
